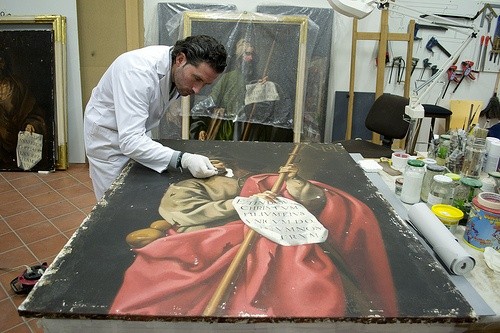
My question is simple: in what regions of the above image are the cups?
[391,152,410,172]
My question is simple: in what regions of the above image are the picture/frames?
[0,12,70,173]
[157,3,334,142]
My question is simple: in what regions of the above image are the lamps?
[328,0,479,119]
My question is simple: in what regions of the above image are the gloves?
[181,152,218,179]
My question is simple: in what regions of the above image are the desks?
[18,139,500,333]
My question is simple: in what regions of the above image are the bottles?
[445,173,461,205]
[420,164,446,203]
[461,135,487,180]
[430,203,465,235]
[462,192,500,253]
[427,174,453,210]
[485,140,500,173]
[481,137,500,172]
[488,171,500,194]
[400,159,425,205]
[451,178,484,226]
[437,134,452,159]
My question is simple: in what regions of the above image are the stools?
[404,104,453,156]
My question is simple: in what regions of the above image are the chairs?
[333,94,409,159]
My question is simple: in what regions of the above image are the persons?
[83,34,227,203]
